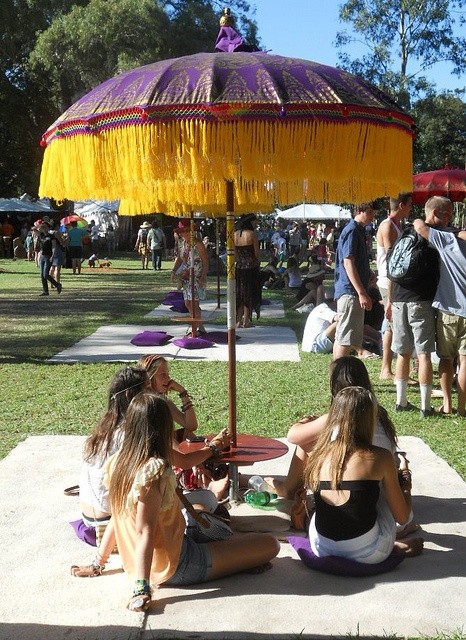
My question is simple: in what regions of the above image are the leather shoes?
[420,407,435,417]
[396,400,421,411]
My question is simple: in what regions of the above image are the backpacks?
[385,223,437,285]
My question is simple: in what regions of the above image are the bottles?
[244,492,281,506]
[247,474,285,501]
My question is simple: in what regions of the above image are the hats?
[306,264,327,278]
[34,219,42,227]
[140,221,152,228]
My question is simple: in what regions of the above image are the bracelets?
[398,469,412,489]
[90,551,108,576]
[181,401,193,411]
[203,439,220,456]
[131,578,151,599]
[179,390,188,398]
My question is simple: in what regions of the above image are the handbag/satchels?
[96,524,119,554]
[158,241,163,247]
[291,487,309,530]
[193,512,233,541]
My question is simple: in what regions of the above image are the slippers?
[439,405,450,414]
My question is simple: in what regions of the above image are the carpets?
[0,433,466,639]
[143,299,285,319]
[48,324,301,363]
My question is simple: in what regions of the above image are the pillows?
[166,292,184,300]
[68,519,96,547]
[172,338,214,349]
[172,300,188,313]
[130,331,174,346]
[202,332,242,343]
[286,536,407,577]
[162,297,182,305]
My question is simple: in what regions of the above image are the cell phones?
[393,452,406,467]
[186,436,205,443]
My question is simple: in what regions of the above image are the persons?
[413,219,465,416]
[135,355,198,432]
[376,192,418,386]
[262,356,397,501]
[389,195,452,417]
[68,221,88,275]
[233,213,264,328]
[88,251,98,268]
[104,223,117,257]
[281,257,303,289]
[257,220,338,261]
[70,391,280,612]
[292,264,325,309]
[304,386,424,567]
[301,287,382,355]
[260,257,280,290]
[170,219,209,338]
[134,222,151,269]
[88,220,99,251]
[79,368,234,545]
[331,201,378,358]
[25,216,67,296]
[147,222,166,270]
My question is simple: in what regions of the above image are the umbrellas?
[39,15,418,448]
[411,165,466,205]
[276,203,352,227]
[115,199,274,337]
[59,214,87,232]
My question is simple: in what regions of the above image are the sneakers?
[39,292,49,296]
[57,283,62,293]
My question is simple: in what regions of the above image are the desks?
[176,429,289,504]
[171,317,208,339]
